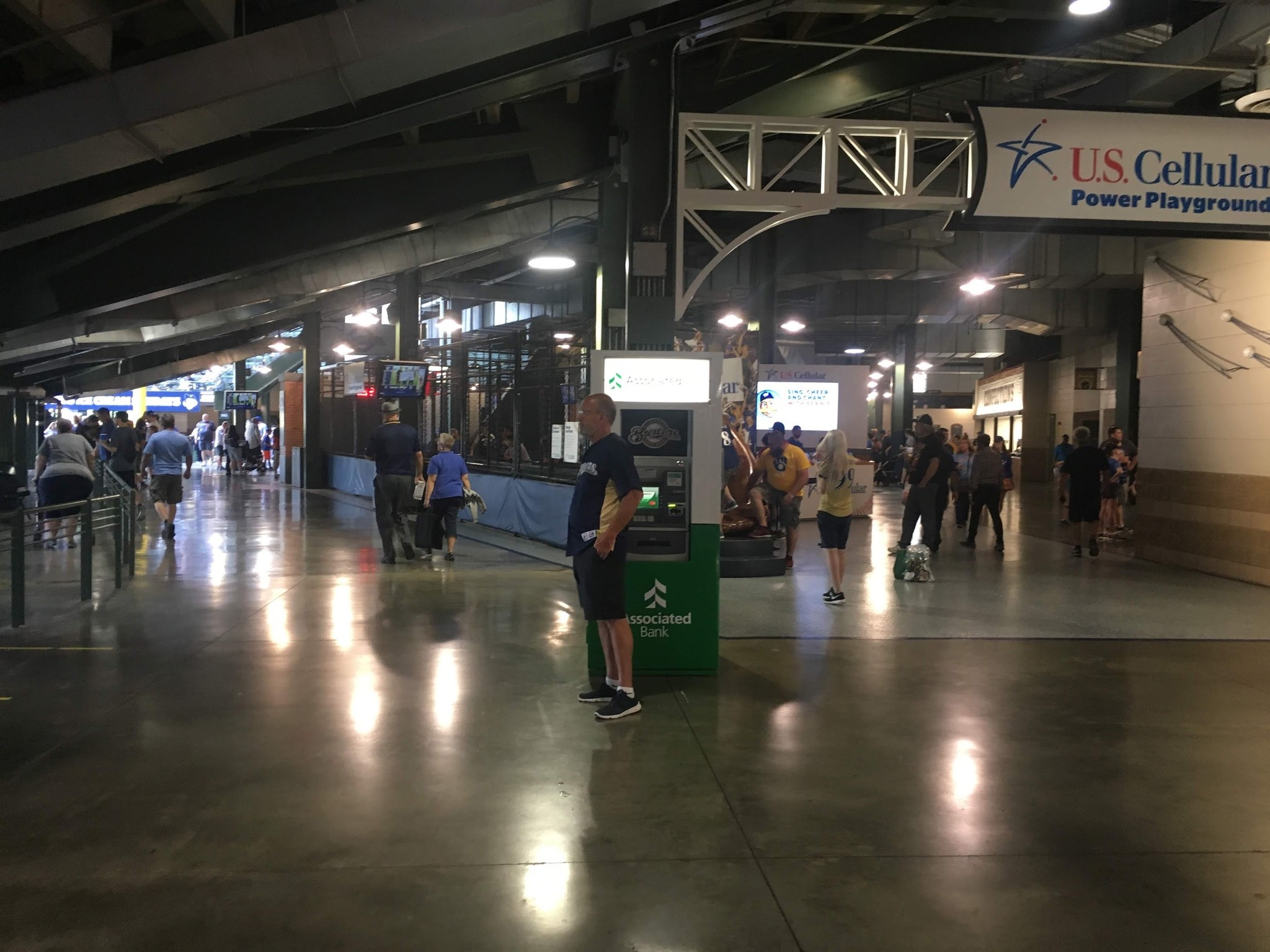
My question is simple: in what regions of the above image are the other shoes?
[136,503,147,521]
[67,537,77,549]
[199,458,279,479]
[887,544,903,555]
[444,550,455,560]
[723,499,738,513]
[1058,517,1134,557]
[420,549,433,559]
[49,538,57,546]
[955,519,1005,551]
[402,542,415,560]
[381,555,396,564]
[161,519,176,539]
[772,528,786,540]
[138,482,149,494]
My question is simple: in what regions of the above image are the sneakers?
[748,525,773,539]
[823,587,846,604]
[785,557,793,568]
[577,681,644,720]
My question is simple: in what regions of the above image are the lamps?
[843,282,866,356]
[320,326,355,356]
[876,283,896,368]
[958,234,997,298]
[915,359,935,372]
[255,354,272,376]
[528,197,604,273]
[779,314,807,331]
[716,288,746,332]
[267,323,304,353]
[350,286,400,329]
[419,290,464,335]
[867,371,885,381]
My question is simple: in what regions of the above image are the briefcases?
[1001,478,1015,492]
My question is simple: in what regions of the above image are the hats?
[378,401,401,415]
[769,422,785,433]
[910,413,934,426]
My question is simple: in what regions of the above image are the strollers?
[240,441,268,475]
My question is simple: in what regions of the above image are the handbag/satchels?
[413,505,445,551]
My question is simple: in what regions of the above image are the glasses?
[578,408,600,416]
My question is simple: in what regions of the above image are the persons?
[396,366,417,386]
[31,400,530,565]
[568,393,645,720]
[722,405,1138,604]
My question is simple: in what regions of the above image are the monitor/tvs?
[635,485,664,511]
[375,359,426,396]
[224,389,260,410]
